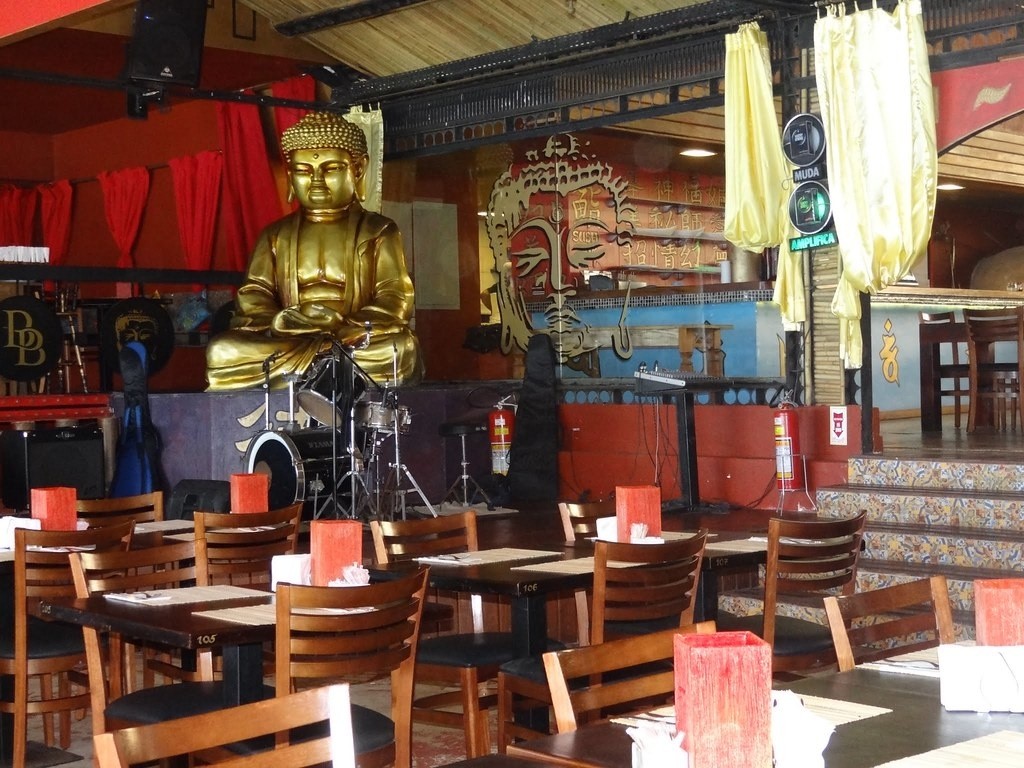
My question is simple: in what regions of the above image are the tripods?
[313,336,438,524]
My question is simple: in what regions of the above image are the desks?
[35,586,454,709]
[140,520,309,676]
[360,533,866,742]
[436,636,1022,768]
[918,323,1024,433]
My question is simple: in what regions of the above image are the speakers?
[127,0,209,87]
[169,478,232,521]
[0,425,105,512]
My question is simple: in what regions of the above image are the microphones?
[379,383,390,417]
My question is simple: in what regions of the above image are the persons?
[201,114,424,394]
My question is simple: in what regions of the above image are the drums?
[241,424,365,522]
[355,400,413,437]
[297,357,370,428]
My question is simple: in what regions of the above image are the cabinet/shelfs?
[607,196,725,275]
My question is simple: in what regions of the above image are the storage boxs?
[672,630,773,768]
[231,473,269,513]
[973,578,1024,645]
[616,485,662,543]
[31,487,77,531]
[310,519,363,586]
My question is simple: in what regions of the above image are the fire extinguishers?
[768,384,805,491]
[488,395,518,477]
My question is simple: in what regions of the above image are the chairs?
[1,490,958,768]
[918,308,1024,433]
